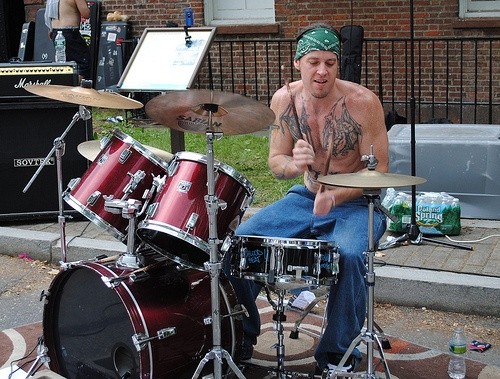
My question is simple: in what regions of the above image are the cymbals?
[23,83,144,109]
[144,88,276,134]
[316,169,426,188]
[77,139,174,164]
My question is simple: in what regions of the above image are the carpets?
[1,296,499,379]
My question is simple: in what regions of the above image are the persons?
[48,0,92,82]
[224,22,387,379]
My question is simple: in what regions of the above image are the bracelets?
[282,159,293,178]
[329,191,336,209]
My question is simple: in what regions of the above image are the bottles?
[447,327,466,379]
[54,31,66,63]
[185,4,193,26]
[387,191,461,235]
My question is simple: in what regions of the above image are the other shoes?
[236,345,254,360]
[322,360,354,379]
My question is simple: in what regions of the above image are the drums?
[136,150,256,273]
[227,233,341,286]
[62,128,168,247]
[37,250,245,379]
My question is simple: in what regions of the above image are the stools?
[289,242,392,349]
